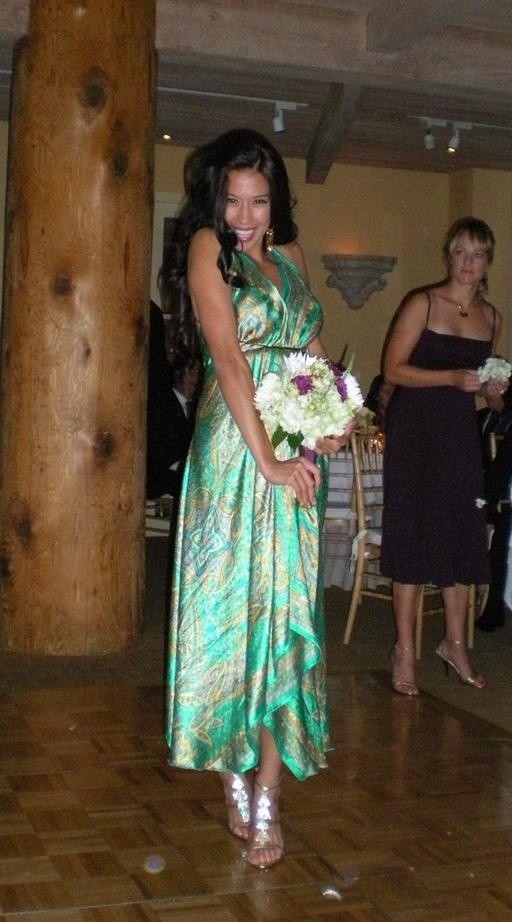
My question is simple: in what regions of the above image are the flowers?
[477,356,512,387]
[252,349,366,468]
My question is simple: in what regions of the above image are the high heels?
[435,637,489,690]
[389,642,421,698]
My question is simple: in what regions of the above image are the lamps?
[321,250,398,312]
[421,118,461,152]
[270,101,297,134]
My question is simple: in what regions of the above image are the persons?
[366,217,512,696]
[366,374,396,433]
[165,128,356,869]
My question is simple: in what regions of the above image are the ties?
[185,400,196,420]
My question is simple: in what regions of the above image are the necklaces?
[459,305,468,317]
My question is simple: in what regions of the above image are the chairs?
[341,411,486,662]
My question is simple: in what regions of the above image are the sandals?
[217,770,255,842]
[246,778,284,871]
